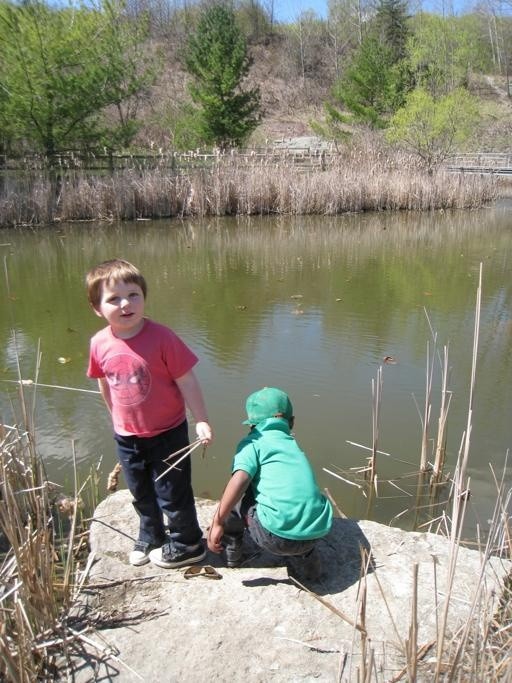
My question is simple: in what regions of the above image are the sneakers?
[149,539,207,569]
[128,538,155,566]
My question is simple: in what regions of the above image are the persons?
[85,257,214,569]
[206,385,333,576]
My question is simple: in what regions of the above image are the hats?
[239,386,293,425]
